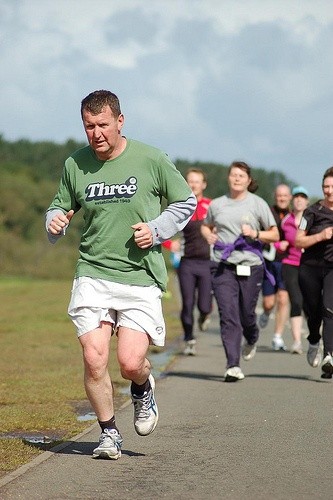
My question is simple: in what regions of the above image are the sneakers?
[92,428,123,460]
[307,343,322,368]
[242,336,256,361]
[321,351,333,379]
[130,374,159,436]
[224,366,244,382]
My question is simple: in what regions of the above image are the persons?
[260,183,293,351]
[201,162,279,381]
[296,166,333,378]
[275,187,311,354]
[44,91,197,458]
[161,168,213,356]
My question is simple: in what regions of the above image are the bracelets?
[256,229,259,239]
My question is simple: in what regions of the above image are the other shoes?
[292,343,303,354]
[259,312,269,328]
[198,313,210,332]
[272,337,287,351]
[185,339,196,355]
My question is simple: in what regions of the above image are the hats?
[292,186,309,198]
